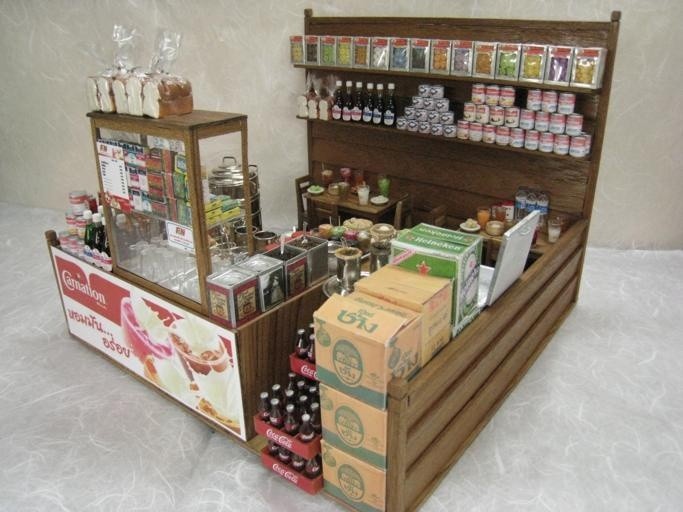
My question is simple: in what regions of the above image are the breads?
[86,67,194,119]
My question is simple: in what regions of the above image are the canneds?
[396,83,591,158]
[58,190,87,259]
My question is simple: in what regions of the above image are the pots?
[208,155,263,232]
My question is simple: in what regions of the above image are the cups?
[234,225,278,251]
[357,185,370,206]
[377,178,391,197]
[476,207,490,231]
[319,167,364,203]
[334,246,363,296]
[167,322,236,423]
[547,219,564,244]
[126,240,232,303]
[117,296,174,376]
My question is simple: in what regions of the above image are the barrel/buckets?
[208,155,263,232]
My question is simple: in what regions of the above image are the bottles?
[257,372,322,473]
[330,79,397,126]
[369,222,396,273]
[514,185,550,234]
[294,322,317,364]
[491,199,515,223]
[83,204,133,273]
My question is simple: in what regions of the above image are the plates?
[459,222,481,233]
[371,196,389,206]
[306,187,325,195]
